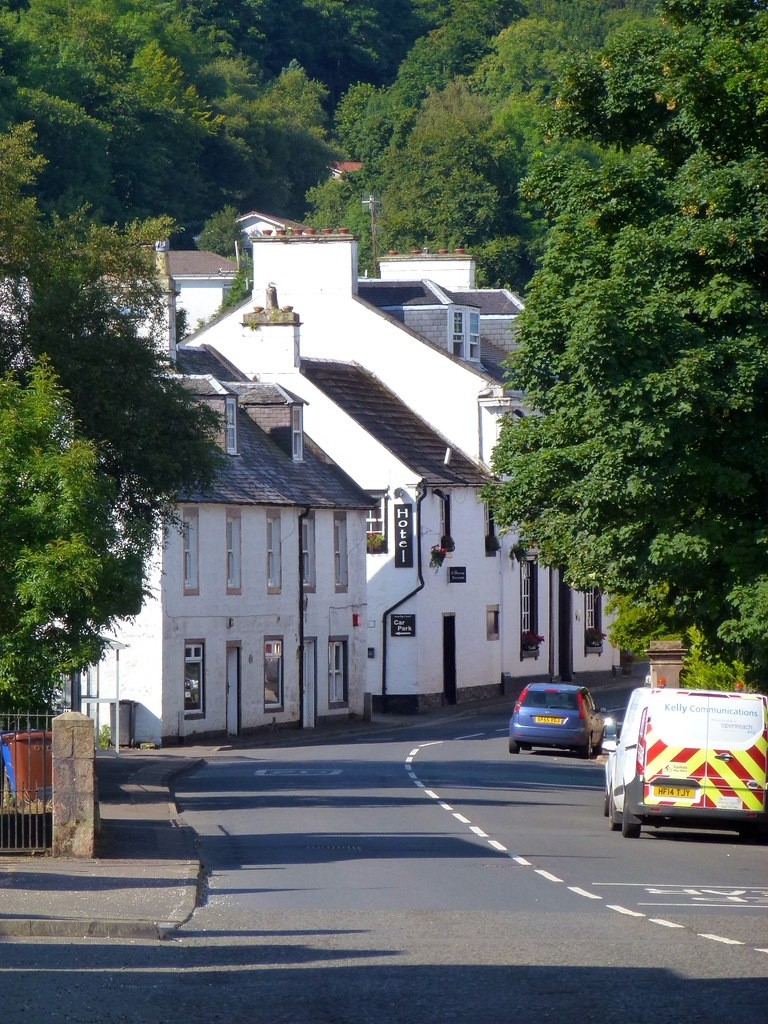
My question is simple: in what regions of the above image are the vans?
[604,680,768,846]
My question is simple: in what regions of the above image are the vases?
[282,306,293,312]
[438,249,448,254]
[262,230,273,236]
[292,229,304,235]
[306,229,316,235]
[410,250,421,254]
[388,251,399,255]
[528,646,536,650]
[322,229,333,234]
[338,228,349,234]
[253,307,264,313]
[454,249,466,254]
[431,552,446,567]
[276,230,286,236]
[591,641,599,646]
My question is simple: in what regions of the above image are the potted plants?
[366,532,387,557]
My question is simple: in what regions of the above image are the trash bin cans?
[2,731,52,802]
[107,700,138,749]
[0,729,41,798]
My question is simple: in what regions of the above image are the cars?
[184,664,199,703]
[509,682,608,758]
[601,740,618,819]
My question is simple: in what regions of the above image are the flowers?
[428,544,447,576]
[586,628,607,647]
[522,631,545,651]
